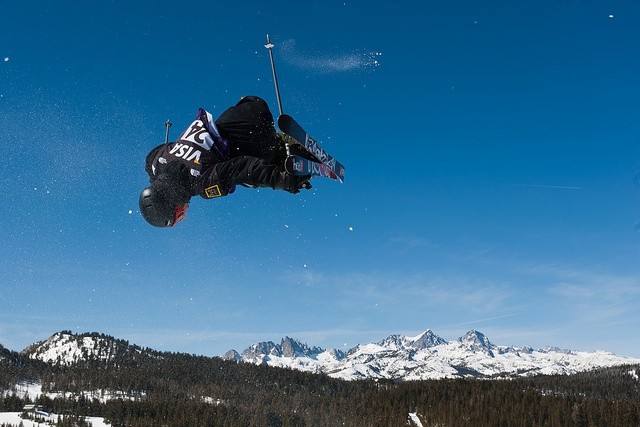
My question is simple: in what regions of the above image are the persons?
[140,96,314,227]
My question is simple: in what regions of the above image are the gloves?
[276,165,299,193]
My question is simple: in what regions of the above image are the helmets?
[139,189,175,227]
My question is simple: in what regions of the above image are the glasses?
[175,204,185,222]
